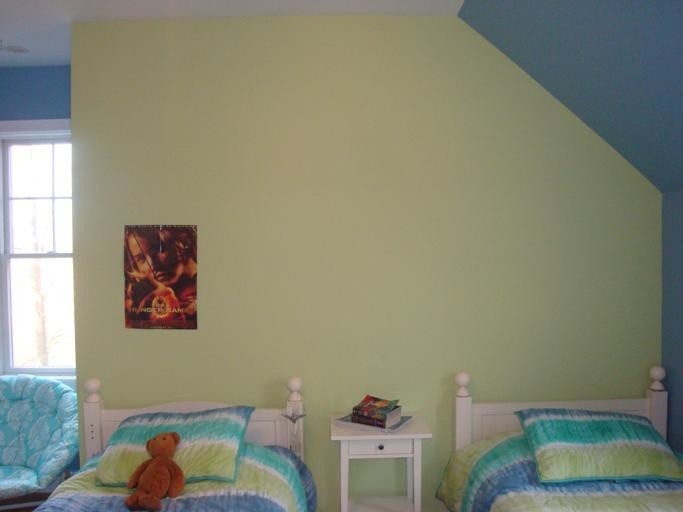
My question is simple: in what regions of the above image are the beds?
[32,376,307,512]
[453,366,683,512]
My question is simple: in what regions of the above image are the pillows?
[513,408,683,485]
[95,405,256,487]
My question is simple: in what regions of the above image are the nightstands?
[331,414,432,512]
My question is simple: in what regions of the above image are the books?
[351,411,406,428]
[352,394,402,419]
[335,412,413,432]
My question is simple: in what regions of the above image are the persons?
[125,227,197,328]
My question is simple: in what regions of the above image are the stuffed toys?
[125,431,185,511]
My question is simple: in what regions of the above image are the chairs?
[0,374,80,511]
